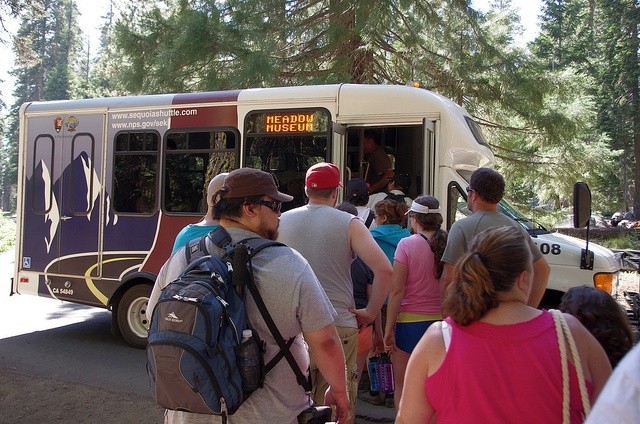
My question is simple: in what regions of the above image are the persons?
[440,168,550,310]
[383,195,450,413]
[365,171,417,209]
[357,130,392,193]
[346,178,377,229]
[557,285,632,370]
[581,341,640,424]
[369,200,412,358]
[273,162,394,424]
[172,172,234,253]
[146,167,352,424]
[324,203,386,423]
[395,224,613,424]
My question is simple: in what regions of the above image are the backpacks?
[383,189,408,203]
[145,236,297,416]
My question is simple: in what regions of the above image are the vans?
[8,83,623,350]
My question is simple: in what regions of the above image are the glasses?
[466,186,474,191]
[372,214,379,218]
[259,199,284,212]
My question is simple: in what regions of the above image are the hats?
[403,199,442,216]
[388,168,412,189]
[345,176,369,196]
[221,167,294,201]
[305,162,343,188]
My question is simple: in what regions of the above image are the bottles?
[238,330,260,392]
[368,354,380,392]
[377,351,395,394]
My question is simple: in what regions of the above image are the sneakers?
[359,390,381,404]
[382,396,394,407]
